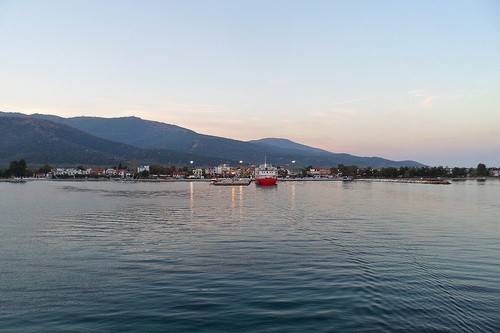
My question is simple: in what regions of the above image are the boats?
[210,175,252,186]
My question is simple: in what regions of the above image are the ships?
[254,155,279,186]
